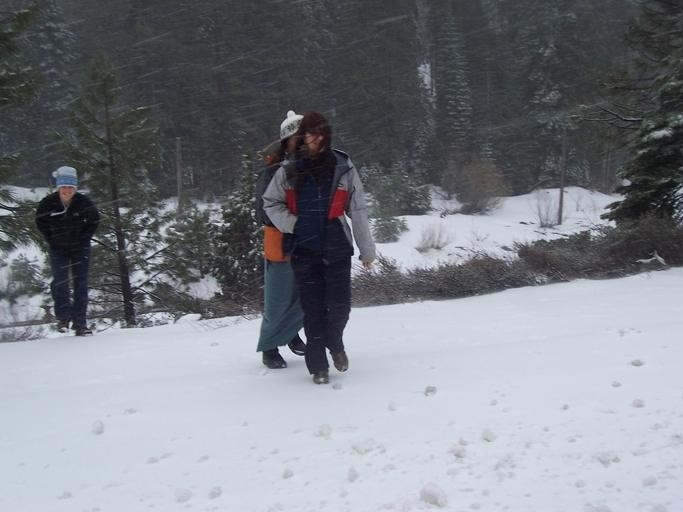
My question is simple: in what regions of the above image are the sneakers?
[76,328,92,335]
[330,349,349,372]
[263,348,287,369]
[313,368,329,384]
[58,320,69,332]
[289,334,306,356]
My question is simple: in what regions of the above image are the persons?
[260,109,378,386]
[251,108,307,370]
[35,166,99,336]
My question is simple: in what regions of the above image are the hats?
[55,165,78,189]
[279,110,304,142]
